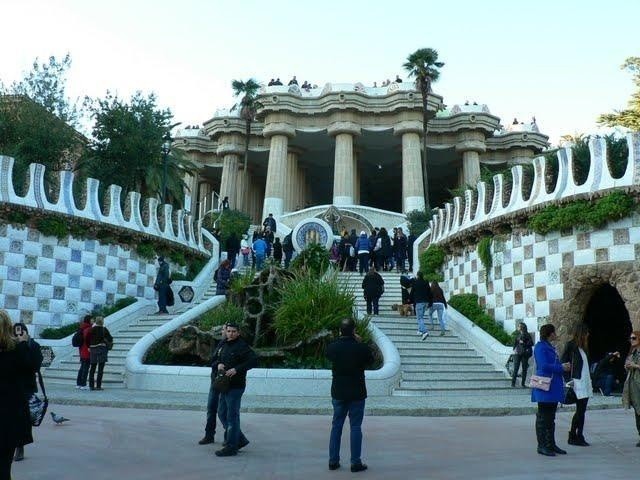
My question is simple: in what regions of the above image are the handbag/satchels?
[621,380,631,413]
[374,238,382,251]
[166,284,174,306]
[528,375,552,392]
[349,246,355,257]
[28,393,48,427]
[103,338,113,350]
[72,328,84,347]
[560,386,578,405]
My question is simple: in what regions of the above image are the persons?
[513,118,517,124]
[474,101,477,105]
[268,75,312,89]
[373,75,402,88]
[465,100,469,105]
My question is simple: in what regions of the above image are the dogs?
[391,303,413,316]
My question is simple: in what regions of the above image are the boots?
[567,430,591,447]
[535,413,566,456]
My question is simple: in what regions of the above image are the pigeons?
[49,411,71,424]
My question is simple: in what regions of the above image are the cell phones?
[14,323,22,337]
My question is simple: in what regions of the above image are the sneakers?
[423,332,429,340]
[198,436,215,444]
[328,463,342,470]
[350,464,368,472]
[417,332,422,336]
[75,385,105,391]
[215,445,239,456]
